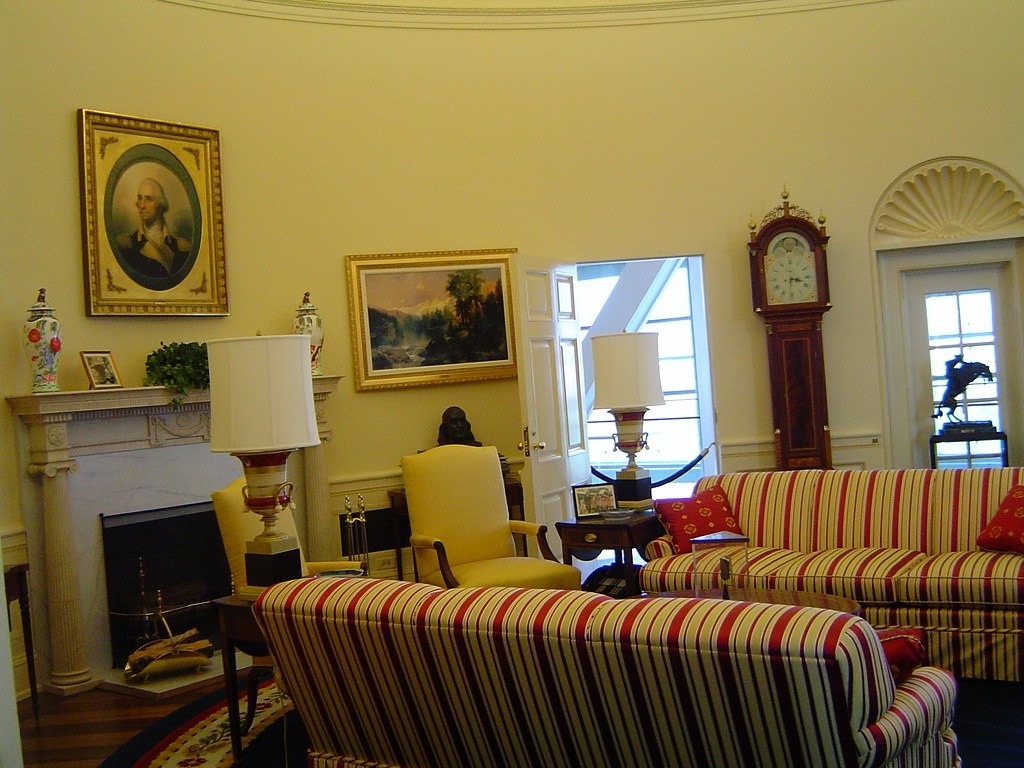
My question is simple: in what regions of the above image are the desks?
[388,479,528,584]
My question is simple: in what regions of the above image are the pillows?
[653,484,744,552]
[976,484,1024,555]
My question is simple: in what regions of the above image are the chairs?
[399,445,580,590]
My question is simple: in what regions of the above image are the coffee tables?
[626,587,861,616]
[213,594,273,768]
[555,510,660,579]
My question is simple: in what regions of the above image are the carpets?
[97,667,309,768]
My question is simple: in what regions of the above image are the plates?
[600,509,636,521]
[319,569,365,578]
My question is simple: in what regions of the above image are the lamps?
[206,330,322,587]
[595,331,666,508]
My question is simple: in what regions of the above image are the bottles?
[292,292,326,376]
[22,287,63,393]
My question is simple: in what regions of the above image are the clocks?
[746,183,832,470]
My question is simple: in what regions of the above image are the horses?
[929,361,993,426]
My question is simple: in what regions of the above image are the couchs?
[255,576,961,768]
[640,466,1024,682]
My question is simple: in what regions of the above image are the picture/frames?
[343,248,519,392]
[79,108,229,316]
[571,483,619,519]
[79,351,124,389]
